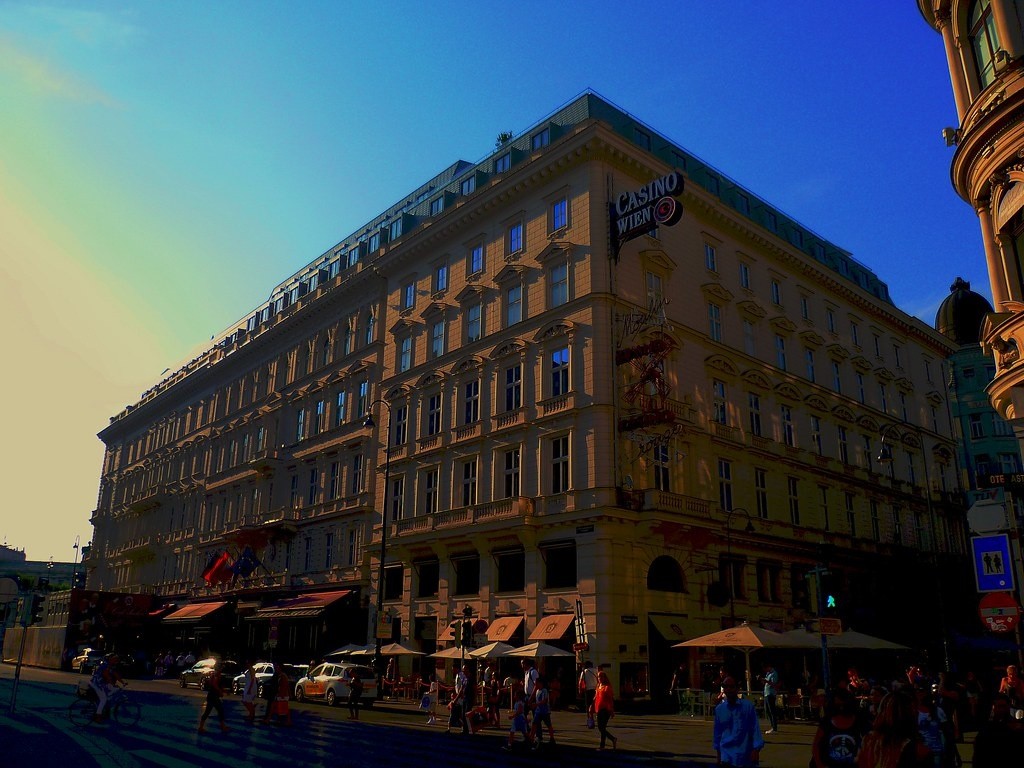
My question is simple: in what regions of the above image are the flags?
[230,547,261,578]
[204,551,237,589]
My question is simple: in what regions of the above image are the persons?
[198,656,292,732]
[670,662,1024,768]
[155,651,195,676]
[92,654,128,724]
[349,658,618,751]
[94,660,104,671]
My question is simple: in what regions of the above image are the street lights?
[362,399,392,703]
[726,508,757,679]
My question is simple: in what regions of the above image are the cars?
[179,658,238,693]
[294,662,378,710]
[232,662,300,699]
[72,647,104,674]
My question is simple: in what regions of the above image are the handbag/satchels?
[586,708,596,730]
[577,678,587,690]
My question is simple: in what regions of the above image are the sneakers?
[532,740,539,750]
[502,746,513,751]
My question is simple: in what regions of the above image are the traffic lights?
[822,592,839,611]
[19,594,46,627]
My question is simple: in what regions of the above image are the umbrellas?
[428,643,575,678]
[669,618,910,698]
[326,642,426,697]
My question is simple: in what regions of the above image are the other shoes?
[96,718,104,724]
[426,717,437,725]
[596,746,607,752]
[347,715,358,720]
[612,737,617,751]
[246,713,293,727]
[765,728,779,735]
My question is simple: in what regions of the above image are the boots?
[219,721,230,732]
[197,720,207,733]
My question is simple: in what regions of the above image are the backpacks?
[537,672,542,683]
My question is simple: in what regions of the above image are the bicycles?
[68,684,143,726]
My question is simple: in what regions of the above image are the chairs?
[696,692,803,721]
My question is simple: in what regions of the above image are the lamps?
[994,50,1015,71]
[942,127,961,146]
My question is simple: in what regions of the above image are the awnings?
[148,594,227,619]
[438,612,575,641]
[245,591,351,618]
[648,614,794,639]
